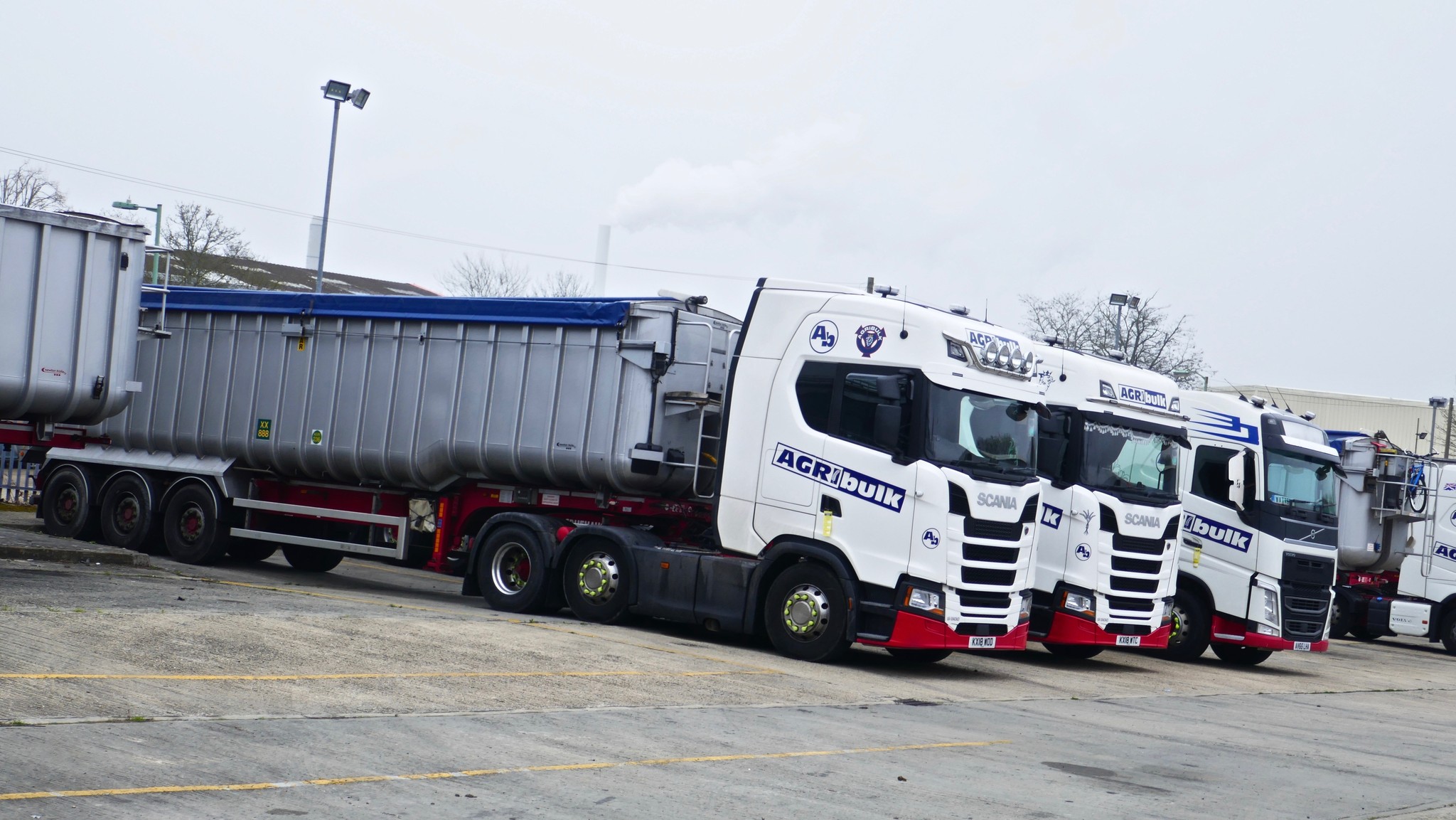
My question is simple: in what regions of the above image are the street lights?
[1108,293,1141,351]
[314,79,370,293]
[1427,396,1449,461]
[111,201,162,283]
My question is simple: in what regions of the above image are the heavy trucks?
[23,273,1456,668]
[0,204,152,452]
